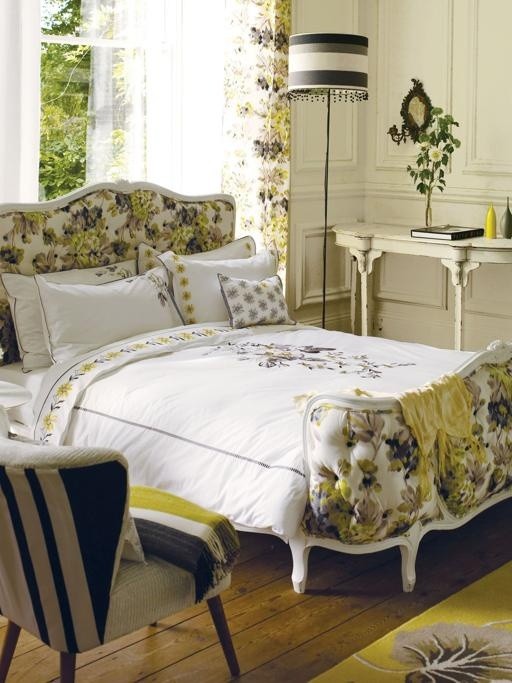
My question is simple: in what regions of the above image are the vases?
[424,192,432,228]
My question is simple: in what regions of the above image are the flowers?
[406,107,462,225]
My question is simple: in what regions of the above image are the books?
[411,224,486,239]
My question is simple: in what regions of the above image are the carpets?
[307,560,512,681]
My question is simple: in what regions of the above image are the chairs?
[0,433,240,681]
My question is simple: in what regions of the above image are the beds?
[0,178,512,592]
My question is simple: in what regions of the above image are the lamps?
[284,32,369,331]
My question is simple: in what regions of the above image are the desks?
[331,222,511,350]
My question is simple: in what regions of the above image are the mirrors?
[387,77,434,144]
